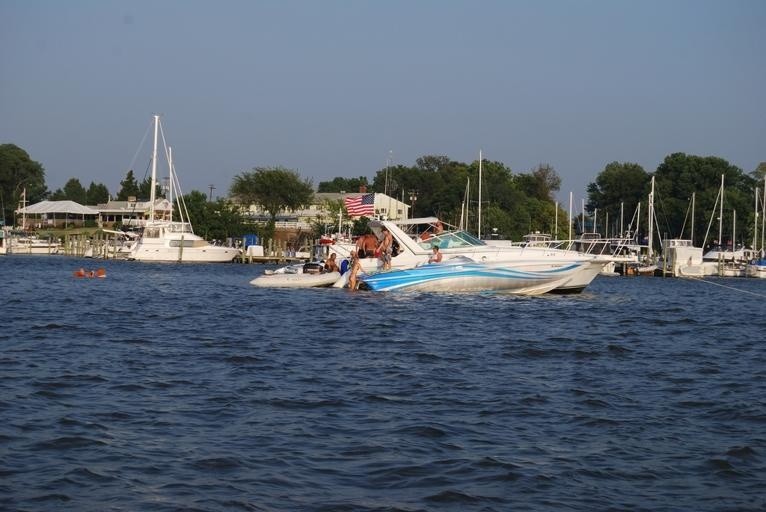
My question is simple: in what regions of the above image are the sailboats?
[1,115,240,262]
[513,173,766,280]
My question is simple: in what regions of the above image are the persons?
[73,267,106,280]
[324,222,443,289]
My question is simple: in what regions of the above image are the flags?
[344,192,375,216]
